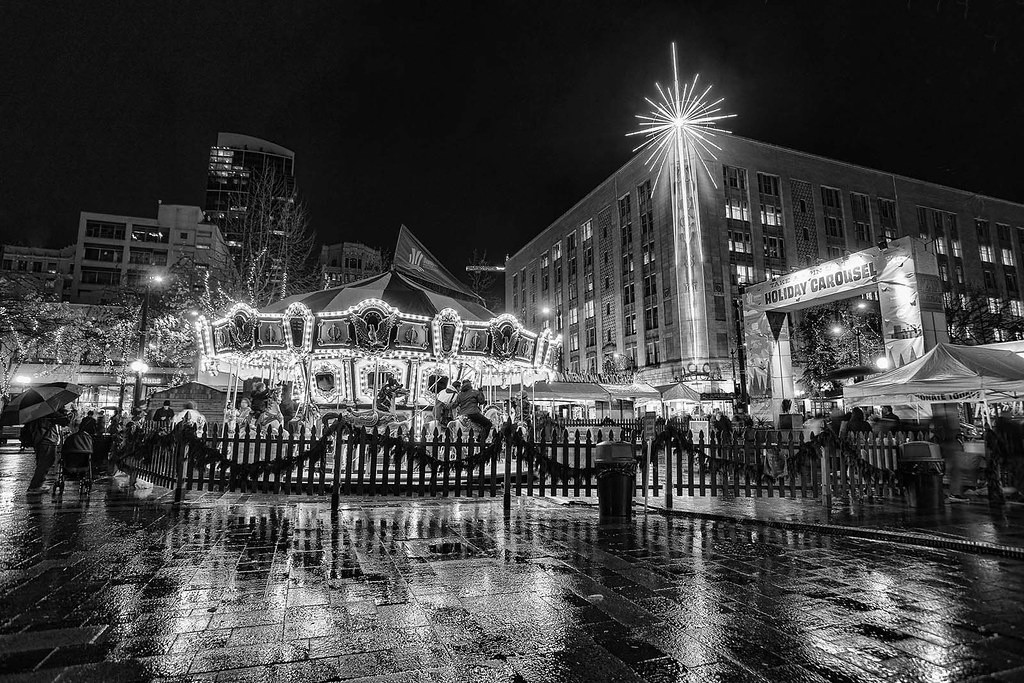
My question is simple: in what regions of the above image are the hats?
[163,400,170,406]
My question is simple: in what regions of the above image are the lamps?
[924,234,937,246]
[739,282,750,288]
[875,235,898,251]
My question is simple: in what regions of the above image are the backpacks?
[20,417,54,448]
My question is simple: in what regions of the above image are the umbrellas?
[100,406,123,410]
[0,381,88,427]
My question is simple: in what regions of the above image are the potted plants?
[779,398,803,429]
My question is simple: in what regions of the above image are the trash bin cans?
[897,442,946,512]
[592,440,637,525]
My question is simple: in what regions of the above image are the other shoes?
[28,484,51,491]
[949,493,969,502]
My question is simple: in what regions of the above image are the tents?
[843,337,1024,425]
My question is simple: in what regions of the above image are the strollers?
[54,425,93,494]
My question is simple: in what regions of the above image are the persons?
[153,399,210,438]
[432,380,461,424]
[79,411,106,437]
[444,378,492,448]
[224,398,252,425]
[928,402,966,498]
[878,406,900,424]
[710,408,733,432]
[840,406,873,435]
[993,410,1024,504]
[24,406,78,492]
[250,382,274,419]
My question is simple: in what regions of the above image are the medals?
[110,409,126,427]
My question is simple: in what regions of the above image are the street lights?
[129,273,162,406]
